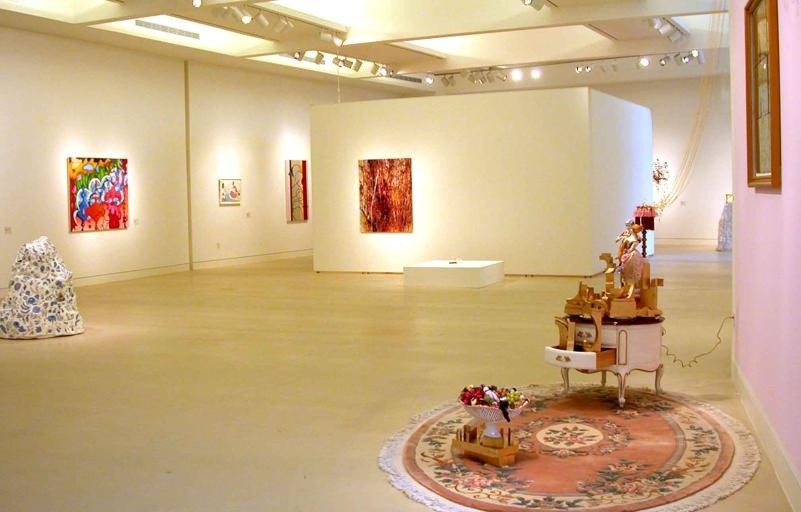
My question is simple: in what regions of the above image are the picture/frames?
[218,178,242,206]
[743,0,782,190]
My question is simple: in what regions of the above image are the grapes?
[459,384,523,408]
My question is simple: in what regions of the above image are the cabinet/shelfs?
[544,317,666,409]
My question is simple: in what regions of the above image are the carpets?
[376,383,762,512]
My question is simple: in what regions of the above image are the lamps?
[441,73,456,87]
[659,51,707,68]
[649,17,684,43]
[524,1,548,11]
[229,5,295,36]
[314,53,324,64]
[633,204,656,262]
[333,55,396,80]
[425,72,434,85]
[469,69,507,87]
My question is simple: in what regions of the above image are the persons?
[615,218,634,286]
[620,223,644,288]
